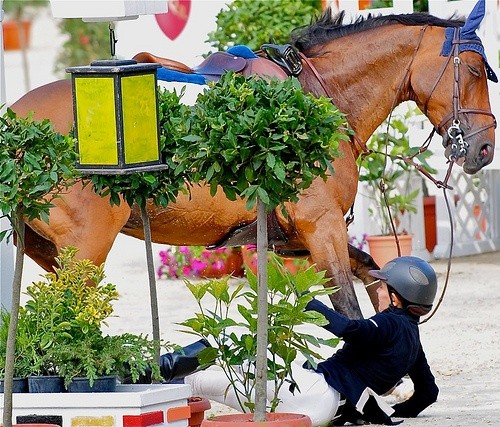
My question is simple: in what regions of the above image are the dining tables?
[0,304,41,392]
[48,331,182,392]
[355,118,437,270]
[174,73,351,427]
[21,245,118,393]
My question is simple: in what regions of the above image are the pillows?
[368,257,437,315]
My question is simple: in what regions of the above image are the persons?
[113,255,440,426]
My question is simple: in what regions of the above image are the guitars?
[60,25,166,179]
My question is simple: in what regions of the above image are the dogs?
[1,0,498,402]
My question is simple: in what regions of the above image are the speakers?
[114,339,215,385]
[160,374,185,384]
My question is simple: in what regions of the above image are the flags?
[226,248,244,278]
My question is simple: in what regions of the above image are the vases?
[156,245,231,281]
